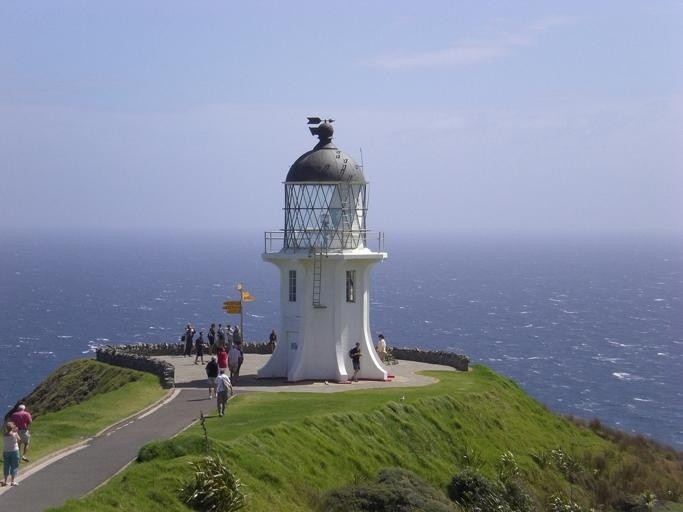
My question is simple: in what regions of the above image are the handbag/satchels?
[222,378,231,393]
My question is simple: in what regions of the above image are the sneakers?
[22,456,29,461]
[219,411,224,417]
[2,480,18,486]
[209,395,217,400]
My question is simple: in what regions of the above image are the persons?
[236,345,244,377]
[179,320,242,365]
[203,356,220,399]
[375,333,387,362]
[347,341,361,383]
[10,403,32,463]
[268,329,279,353]
[0,421,20,487]
[212,369,234,417]
[226,344,239,383]
[215,346,228,375]
